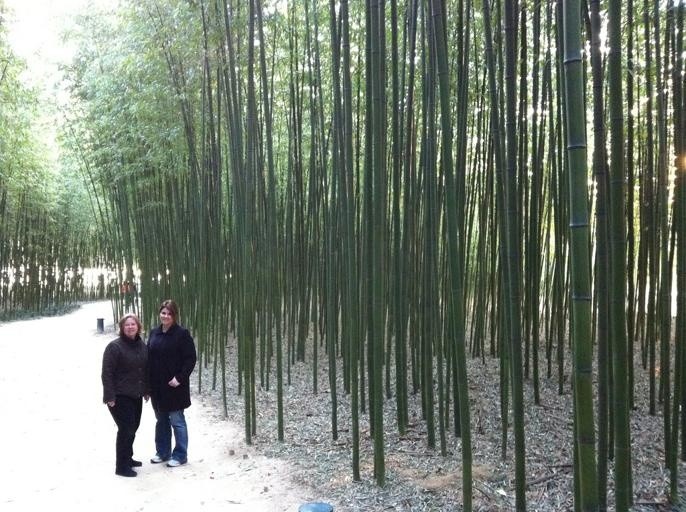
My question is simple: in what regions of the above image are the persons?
[102,313,149,477]
[144,299,197,468]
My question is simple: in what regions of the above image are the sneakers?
[115,459,142,477]
[167,457,182,467]
[150,454,167,463]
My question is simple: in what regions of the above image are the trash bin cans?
[97,319,104,333]
